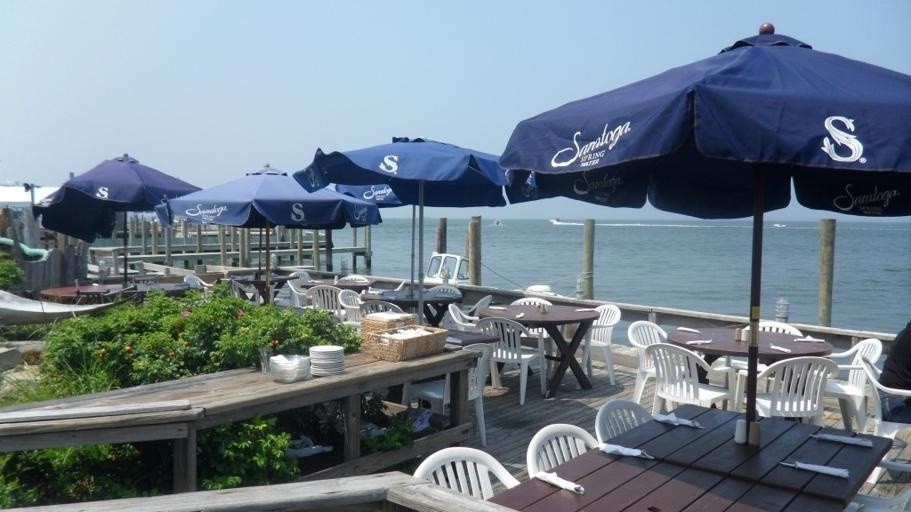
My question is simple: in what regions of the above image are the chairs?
[279,270,621,407]
[75,289,110,305]
[525,423,604,479]
[595,398,655,445]
[132,274,163,283]
[411,445,521,503]
[23,288,38,300]
[103,284,137,303]
[120,290,150,304]
[624,313,911,486]
[850,457,911,512]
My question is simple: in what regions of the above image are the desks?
[486,404,896,511]
[40,283,188,304]
[1,344,484,493]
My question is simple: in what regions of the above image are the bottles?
[736,328,751,342]
[748,421,762,446]
[735,419,749,444]
[536,302,548,314]
[254,272,259,280]
[335,275,339,285]
[405,285,414,297]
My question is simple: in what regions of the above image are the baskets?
[367,324,448,361]
[360,314,417,350]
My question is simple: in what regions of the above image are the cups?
[258,347,272,375]
[163,267,170,275]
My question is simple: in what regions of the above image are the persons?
[877,321,910,425]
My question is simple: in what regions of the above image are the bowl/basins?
[270,353,311,383]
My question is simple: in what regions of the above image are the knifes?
[779,461,796,467]
[808,432,819,438]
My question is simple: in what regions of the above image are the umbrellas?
[335,182,416,314]
[498,22,910,445]
[293,137,507,328]
[154,163,382,306]
[33,153,204,290]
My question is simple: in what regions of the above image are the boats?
[423,251,565,298]
[494,218,500,226]
[771,222,786,229]
[549,220,561,224]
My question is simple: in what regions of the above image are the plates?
[308,345,347,376]
[194,264,206,274]
